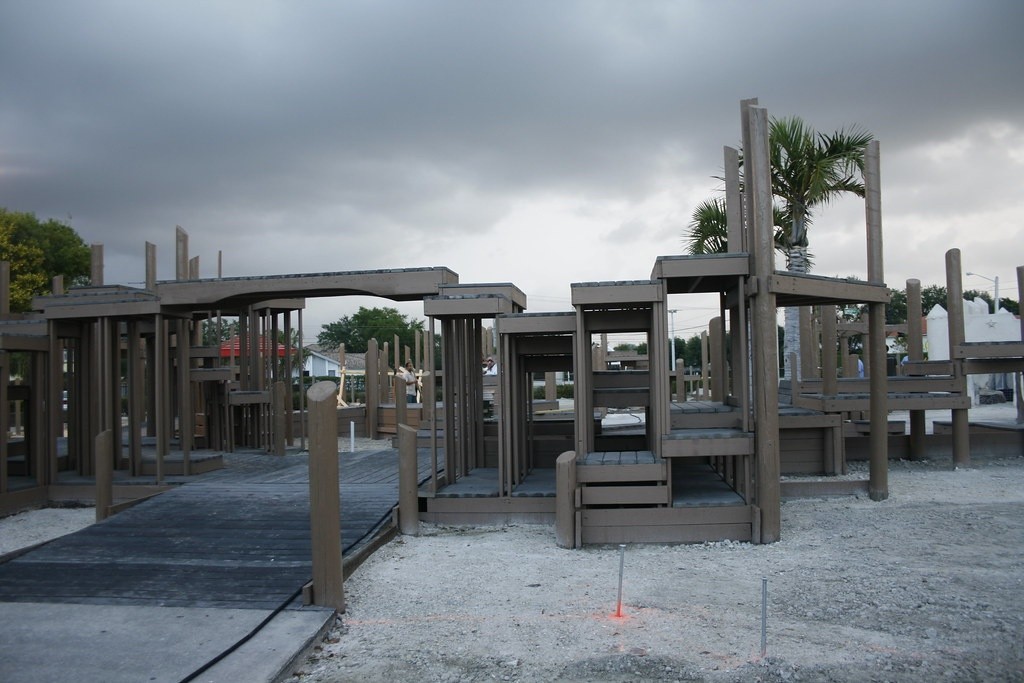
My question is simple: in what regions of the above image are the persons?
[402,361,418,403]
[481,353,497,375]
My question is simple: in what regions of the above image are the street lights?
[966,271,1000,314]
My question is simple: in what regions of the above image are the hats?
[487,357,493,361]
